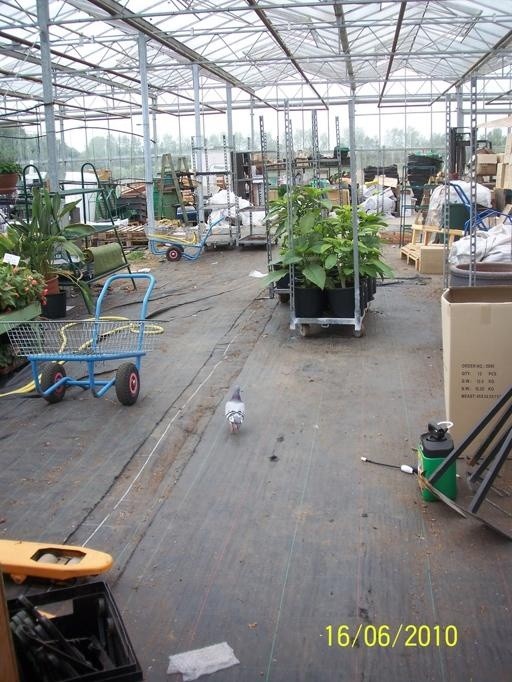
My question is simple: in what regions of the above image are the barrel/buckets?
[417,421,457,501]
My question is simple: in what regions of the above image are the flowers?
[1,260,49,310]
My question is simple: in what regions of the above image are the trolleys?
[143,216,224,262]
[0,274,171,407]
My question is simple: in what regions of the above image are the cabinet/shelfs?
[1,163,138,315]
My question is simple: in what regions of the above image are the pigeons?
[224,386,246,434]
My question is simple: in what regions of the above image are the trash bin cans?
[153,177,177,220]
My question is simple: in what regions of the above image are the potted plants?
[0,179,96,315]
[258,182,394,318]
[0,160,25,194]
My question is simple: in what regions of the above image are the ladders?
[158,153,200,225]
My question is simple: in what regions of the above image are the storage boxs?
[441,285,512,457]
[477,133,511,189]
